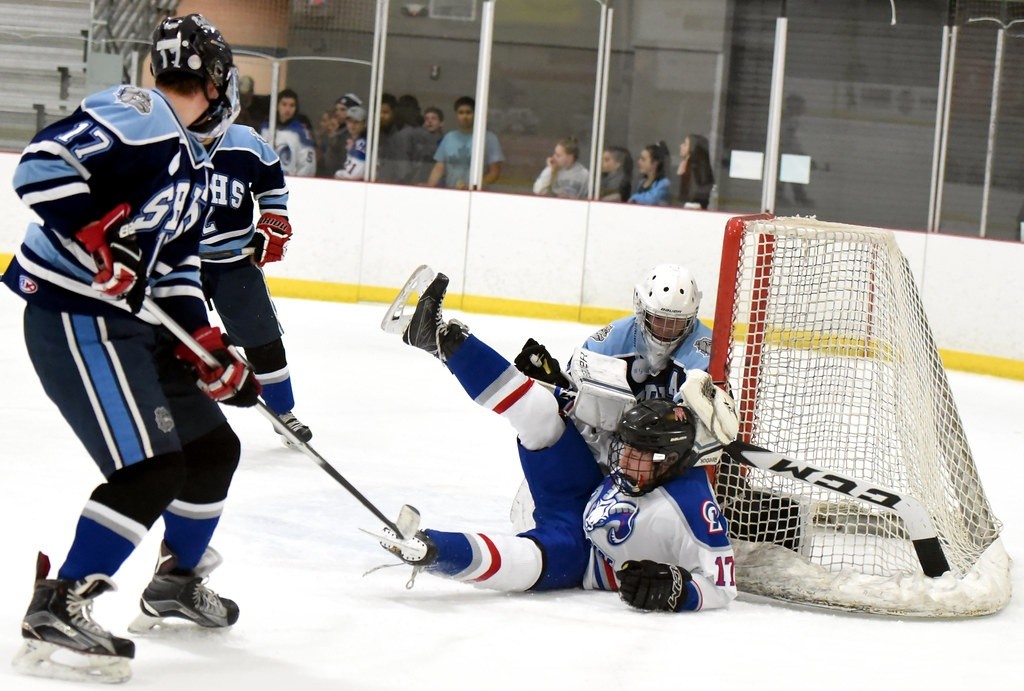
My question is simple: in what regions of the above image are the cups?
[1020,221,1024,243]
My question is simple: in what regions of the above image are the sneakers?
[128,539,239,637]
[13,550,136,684]
[359,526,439,589]
[274,410,313,449]
[380,264,468,363]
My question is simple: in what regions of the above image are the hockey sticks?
[198,247,255,260]
[142,295,420,552]
[530,377,951,579]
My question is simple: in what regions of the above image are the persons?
[774,94,821,209]
[533,135,590,198]
[196,119,315,454]
[1,12,258,686]
[562,264,798,548]
[676,134,716,210]
[627,139,671,205]
[598,147,637,201]
[236,75,508,192]
[357,264,738,613]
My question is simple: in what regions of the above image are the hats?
[344,107,367,122]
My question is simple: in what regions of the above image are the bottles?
[707,184,719,211]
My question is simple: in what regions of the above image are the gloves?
[616,559,692,613]
[174,326,264,408]
[75,204,148,313]
[249,212,291,265]
[514,338,561,384]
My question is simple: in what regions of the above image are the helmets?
[150,13,241,137]
[605,398,695,496]
[633,263,703,370]
